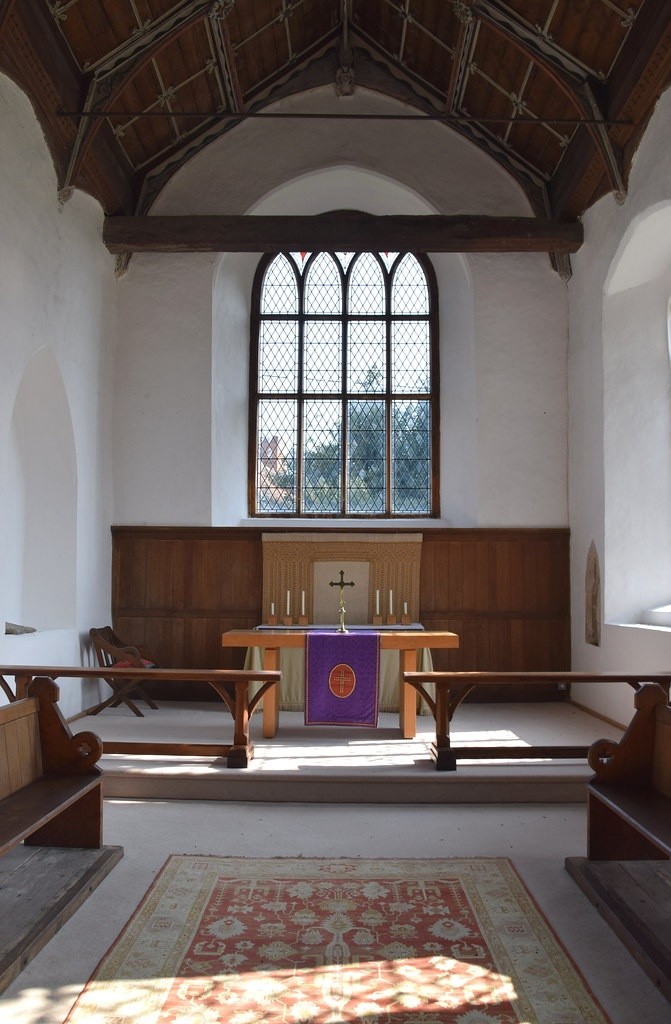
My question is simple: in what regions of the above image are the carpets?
[63,854,613,1024]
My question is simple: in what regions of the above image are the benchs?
[401,672,671,770]
[566,682,671,1001]
[0,665,282,769]
[0,675,125,996]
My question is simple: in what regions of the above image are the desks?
[222,628,459,737]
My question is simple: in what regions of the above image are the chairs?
[89,626,159,716]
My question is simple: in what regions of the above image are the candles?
[376,589,379,614]
[389,590,392,614]
[271,602,274,615]
[287,590,290,615]
[302,590,305,615]
[404,601,407,614]
